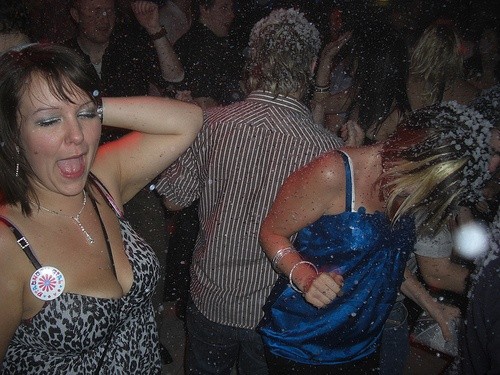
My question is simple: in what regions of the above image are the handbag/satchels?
[409,308,459,356]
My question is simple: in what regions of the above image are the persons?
[0,44,203,374]
[155,9,365,375]
[1,0,500,374]
[257,99,492,375]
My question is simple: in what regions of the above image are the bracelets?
[149,26,167,41]
[272,246,318,293]
[313,80,330,93]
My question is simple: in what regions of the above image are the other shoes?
[157,344,172,364]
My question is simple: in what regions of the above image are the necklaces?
[31,190,96,244]
